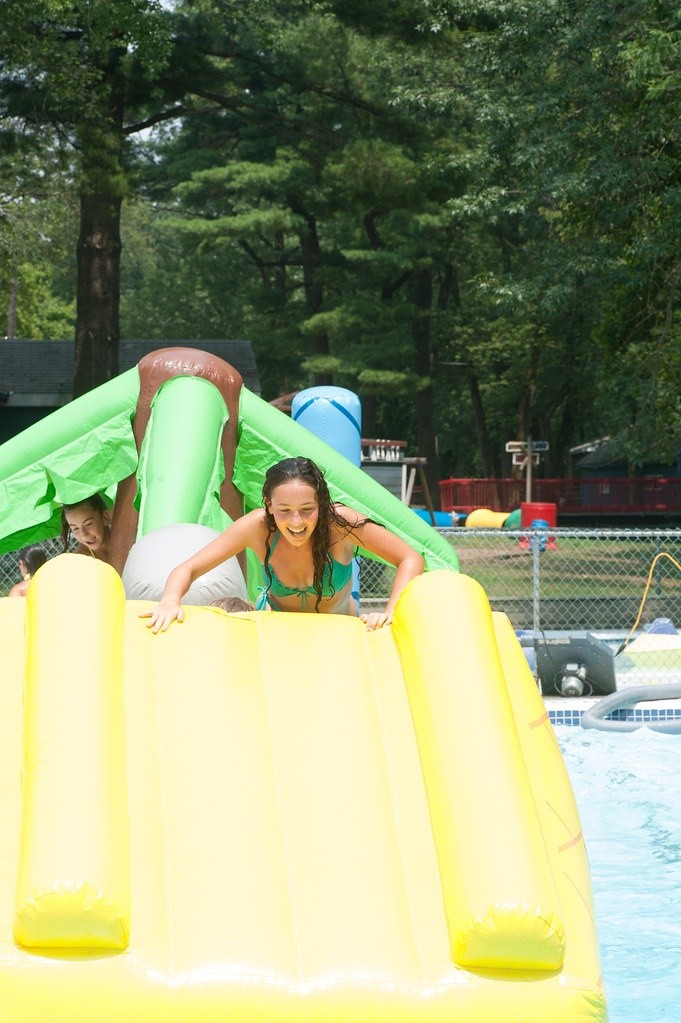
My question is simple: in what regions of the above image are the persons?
[138,456,425,634]
[8,544,47,597]
[61,495,111,562]
